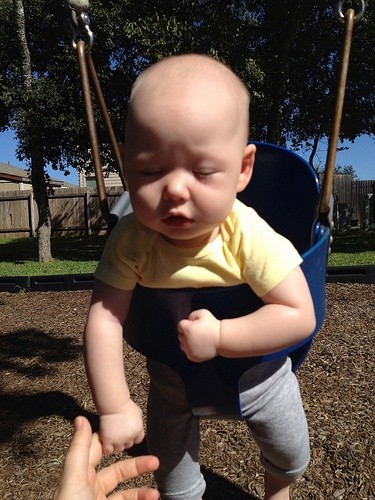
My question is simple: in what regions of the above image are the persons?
[84,54,317,500]
[58,415,159,500]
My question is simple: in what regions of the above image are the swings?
[67,1,368,386]
